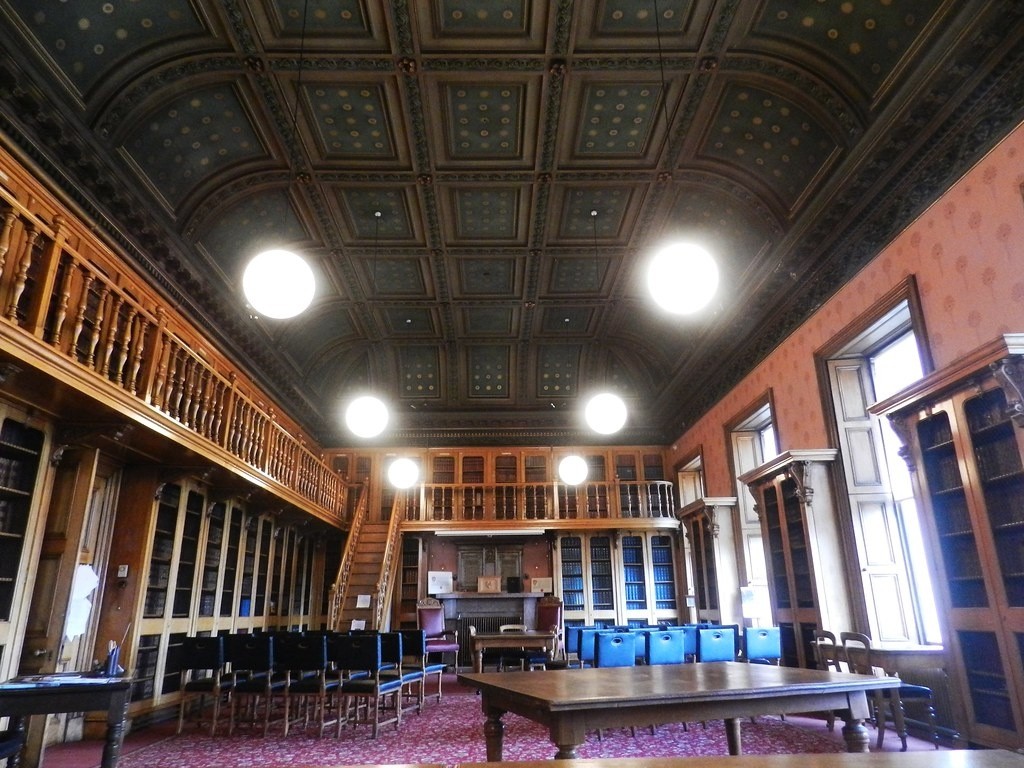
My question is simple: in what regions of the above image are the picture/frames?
[478,576,501,593]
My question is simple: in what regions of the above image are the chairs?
[468,625,503,672]
[604,624,633,633]
[576,628,615,668]
[226,634,298,739]
[841,631,939,750]
[625,628,665,657]
[273,628,443,740]
[643,629,689,735]
[498,625,527,673]
[564,625,597,669]
[705,624,740,662]
[534,594,566,661]
[593,631,636,742]
[696,628,735,730]
[813,629,878,732]
[525,624,557,671]
[666,625,697,663]
[416,597,460,675]
[175,636,246,737]
[743,626,786,725]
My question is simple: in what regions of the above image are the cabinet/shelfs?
[866,330,1024,751]
[552,532,589,661]
[585,531,623,626]
[0,388,55,730]
[391,536,422,668]
[675,496,743,632]
[82,466,315,742]
[737,448,857,721]
[615,530,653,626]
[645,530,683,626]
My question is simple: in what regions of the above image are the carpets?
[92,692,848,768]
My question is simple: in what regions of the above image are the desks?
[810,640,970,751]
[471,631,557,696]
[458,661,901,768]
[459,748,1024,768]
[0,670,138,768]
[436,593,545,674]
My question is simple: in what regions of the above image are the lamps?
[345,213,387,438]
[241,0,315,319]
[559,318,588,487]
[584,210,627,435]
[387,317,420,490]
[645,0,720,314]
